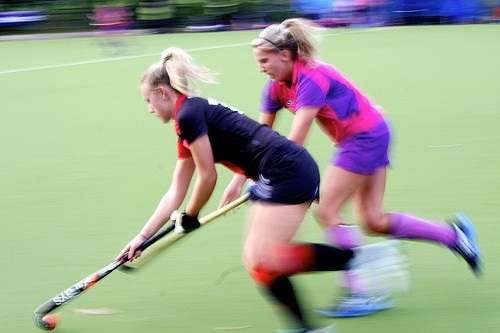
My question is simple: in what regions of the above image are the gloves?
[170,210,199,234]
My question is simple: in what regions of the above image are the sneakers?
[450,214,480,270]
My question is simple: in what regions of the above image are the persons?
[115,45,403,333]
[219,18,483,317]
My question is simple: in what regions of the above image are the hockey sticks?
[117,192,250,276]
[29,221,172,331]
